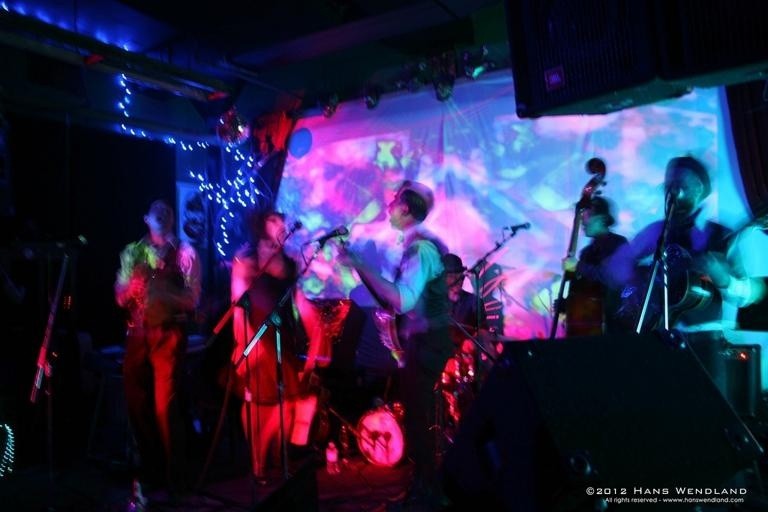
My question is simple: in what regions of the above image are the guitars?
[626,210,768,332]
[341,239,406,368]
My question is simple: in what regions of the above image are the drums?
[357,406,407,466]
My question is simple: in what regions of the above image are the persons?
[230,207,324,477]
[331,178,454,500]
[116,194,205,475]
[601,158,747,409]
[434,247,486,352]
[551,196,632,337]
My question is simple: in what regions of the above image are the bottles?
[326,443,339,474]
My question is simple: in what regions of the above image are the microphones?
[318,224,348,242]
[65,230,90,258]
[669,187,685,200]
[507,222,531,230]
[294,221,302,229]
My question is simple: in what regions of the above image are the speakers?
[438,332,764,512]
[504,0,768,119]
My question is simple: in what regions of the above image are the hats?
[588,196,614,226]
[447,254,467,274]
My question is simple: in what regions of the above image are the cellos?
[565,158,608,339]
